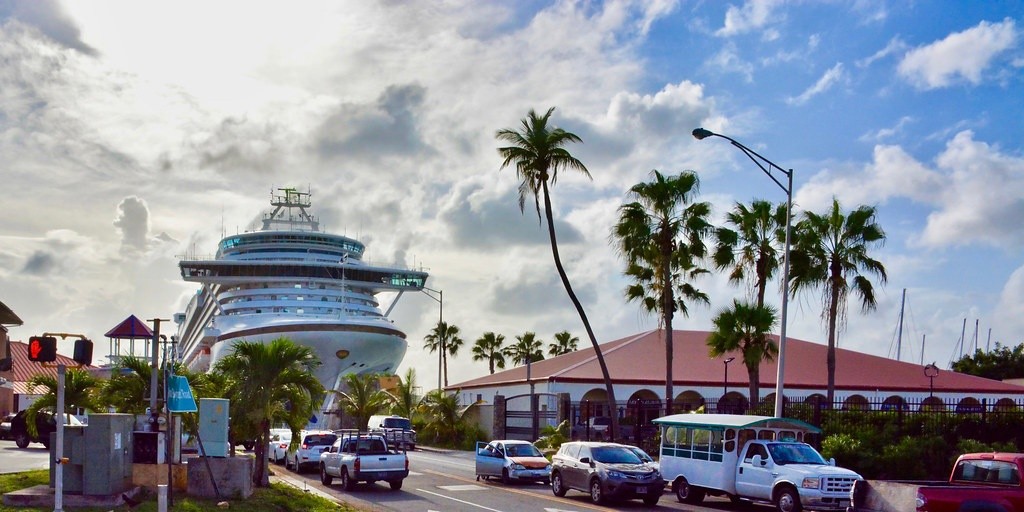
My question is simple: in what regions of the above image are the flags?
[340,252,349,265]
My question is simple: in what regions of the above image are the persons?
[509,447,519,457]
[476,445,494,481]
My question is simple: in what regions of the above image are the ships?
[166,186,427,430]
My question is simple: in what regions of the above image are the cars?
[268,432,303,464]
[551,442,664,507]
[0,414,18,440]
[285,431,338,474]
[11,407,83,450]
[476,440,552,486]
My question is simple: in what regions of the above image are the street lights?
[692,127,794,439]
[380,276,449,389]
[722,357,735,395]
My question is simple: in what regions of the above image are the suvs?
[367,415,417,451]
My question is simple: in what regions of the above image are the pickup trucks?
[320,435,410,492]
[652,413,864,512]
[846,451,1024,512]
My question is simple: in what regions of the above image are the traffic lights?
[28,337,57,364]
